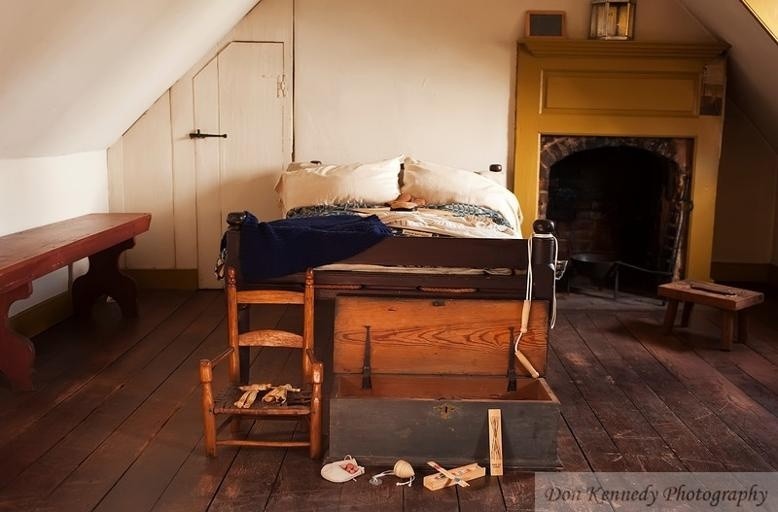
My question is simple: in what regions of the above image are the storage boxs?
[327,297,563,471]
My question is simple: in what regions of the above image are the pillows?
[280,159,522,226]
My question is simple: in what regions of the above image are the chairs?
[197,265,328,460]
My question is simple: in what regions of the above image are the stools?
[662,278,763,351]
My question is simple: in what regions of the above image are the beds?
[226,155,558,381]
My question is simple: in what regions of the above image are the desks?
[0,212,152,391]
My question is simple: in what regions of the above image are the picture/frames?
[587,1,636,40]
[526,10,568,38]
